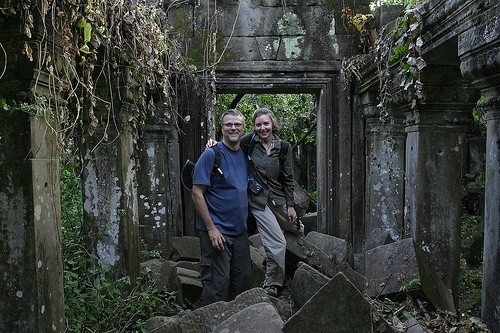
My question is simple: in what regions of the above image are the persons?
[191,109,256,306]
[206,108,305,297]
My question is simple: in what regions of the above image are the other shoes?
[259,282,282,299]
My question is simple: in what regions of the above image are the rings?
[295,216,297,219]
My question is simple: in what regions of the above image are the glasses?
[222,122,244,127]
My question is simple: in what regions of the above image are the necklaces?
[266,137,273,151]
